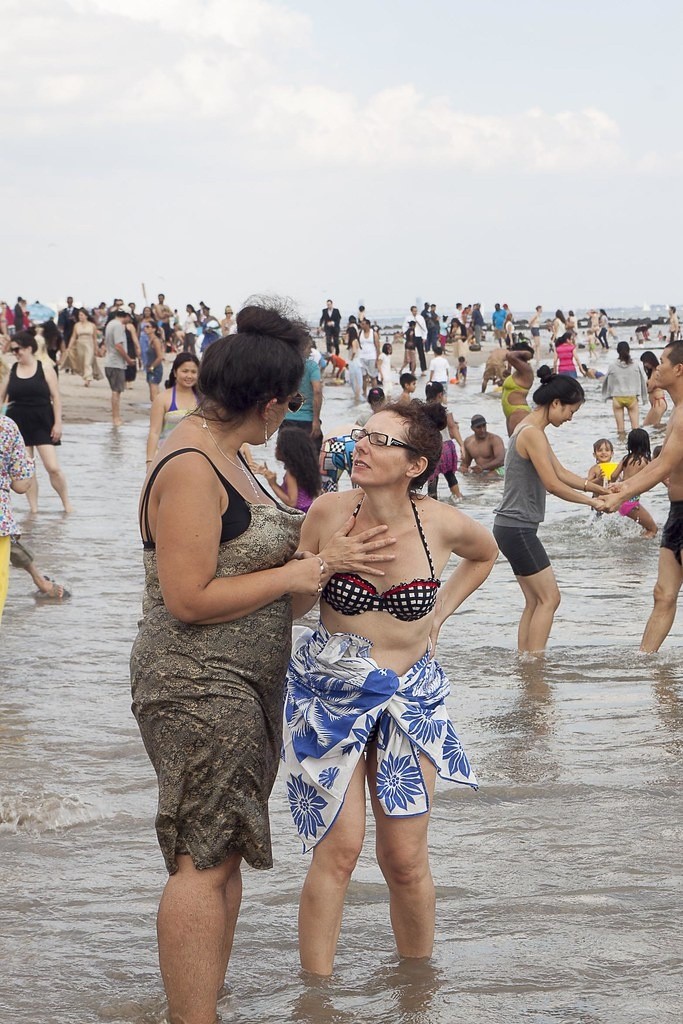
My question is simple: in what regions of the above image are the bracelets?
[583,479,588,492]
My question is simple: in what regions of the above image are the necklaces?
[202,409,260,499]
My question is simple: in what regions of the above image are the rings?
[317,556,323,566]
[317,582,322,593]
[320,565,325,573]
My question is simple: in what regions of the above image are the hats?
[472,414,486,427]
[589,308,596,313]
[118,305,134,319]
[503,304,508,307]
[369,388,384,400]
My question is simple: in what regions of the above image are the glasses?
[10,346,21,352]
[258,392,305,414]
[144,325,151,328]
[226,312,232,314]
[351,429,418,452]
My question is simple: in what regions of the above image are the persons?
[0,294,683,656]
[275,399,496,976]
[128,304,330,1022]
